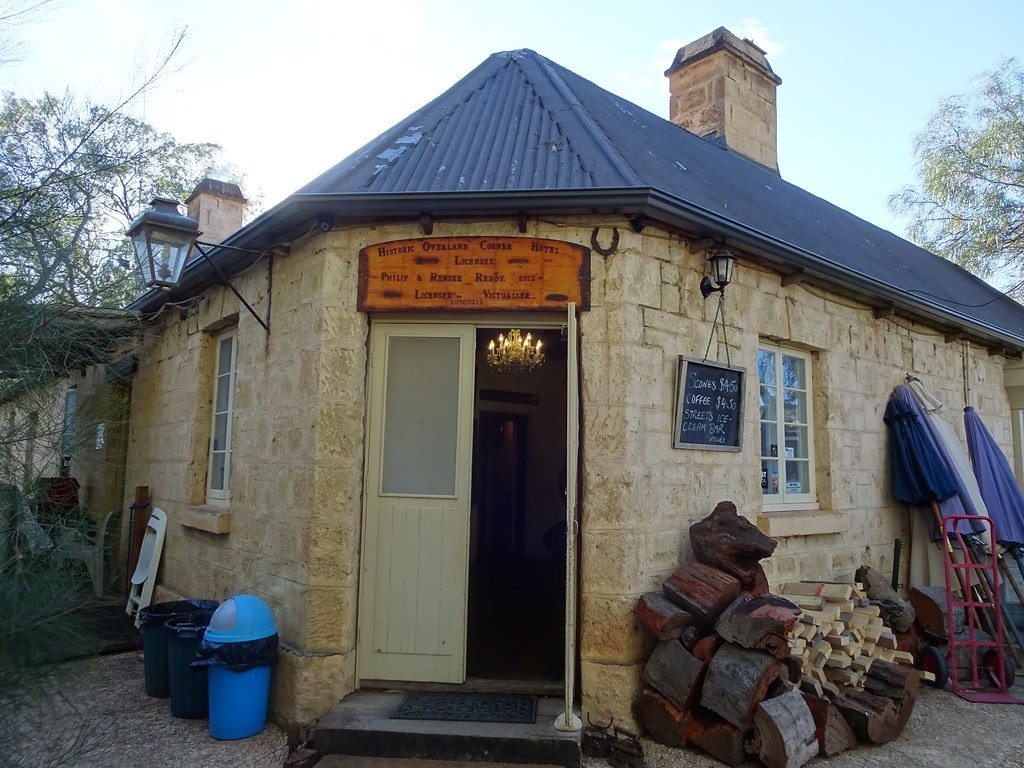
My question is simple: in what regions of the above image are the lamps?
[123,196,272,332]
[486,329,547,374]
[698,247,738,298]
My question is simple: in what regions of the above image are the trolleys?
[918,513,1024,706]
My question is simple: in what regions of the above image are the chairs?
[56,510,121,599]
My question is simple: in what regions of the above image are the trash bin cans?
[165,614,211,719]
[140,600,220,698]
[203,596,280,740]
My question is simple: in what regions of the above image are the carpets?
[389,691,537,726]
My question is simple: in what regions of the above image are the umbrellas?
[883,372,1024,671]
